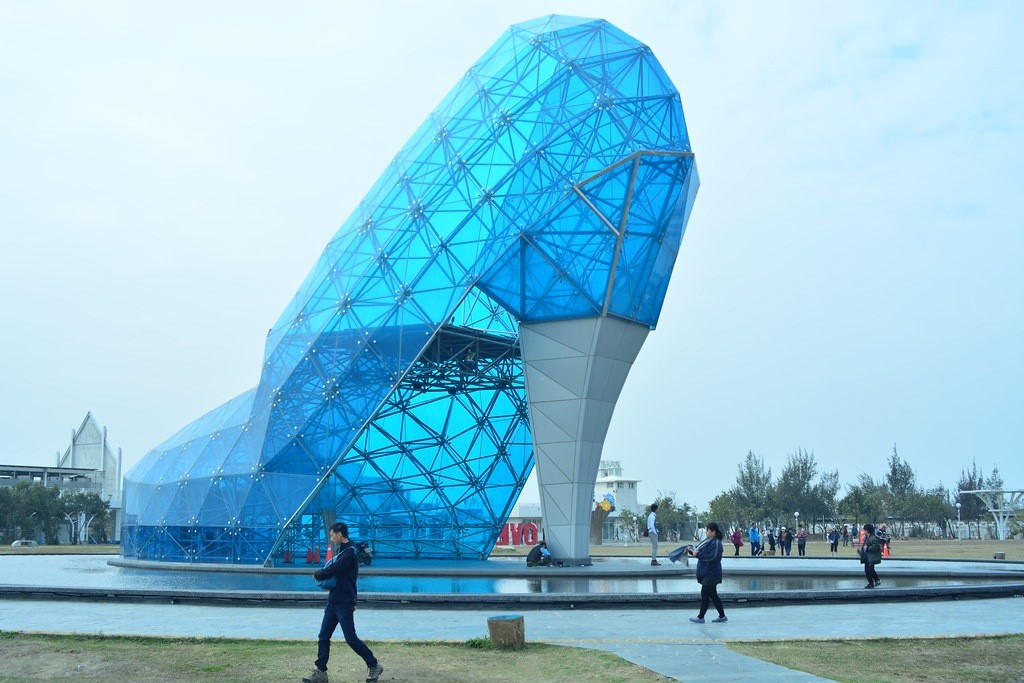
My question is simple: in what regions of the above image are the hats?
[781,527,786,529]
[881,524,888,528]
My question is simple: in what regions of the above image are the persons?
[302,522,384,683]
[688,523,728,623]
[526,542,552,567]
[851,523,858,545]
[843,525,849,547]
[731,526,745,555]
[749,523,807,556]
[828,527,841,555]
[858,524,893,588]
[647,504,661,565]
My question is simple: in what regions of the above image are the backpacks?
[314,547,357,590]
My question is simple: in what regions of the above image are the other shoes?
[874,580,881,586]
[865,583,874,589]
[689,617,705,623]
[712,616,728,622]
[651,560,661,565]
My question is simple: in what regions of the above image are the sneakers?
[302,668,328,683]
[366,662,384,682]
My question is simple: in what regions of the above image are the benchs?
[851,543,889,556]
[11,540,38,547]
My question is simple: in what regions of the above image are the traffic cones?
[303,548,313,565]
[321,543,333,567]
[313,547,321,564]
[883,543,890,558]
[281,548,294,563]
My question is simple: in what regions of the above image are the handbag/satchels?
[738,541,743,546]
[644,529,649,536]
[830,540,834,543]
[853,536,859,543]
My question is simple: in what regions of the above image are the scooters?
[335,541,371,565]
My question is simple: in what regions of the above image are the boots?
[834,552,837,557]
[830,551,833,556]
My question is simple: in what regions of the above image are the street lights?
[633,516,637,543]
[794,511,799,534]
[956,503,961,541]
